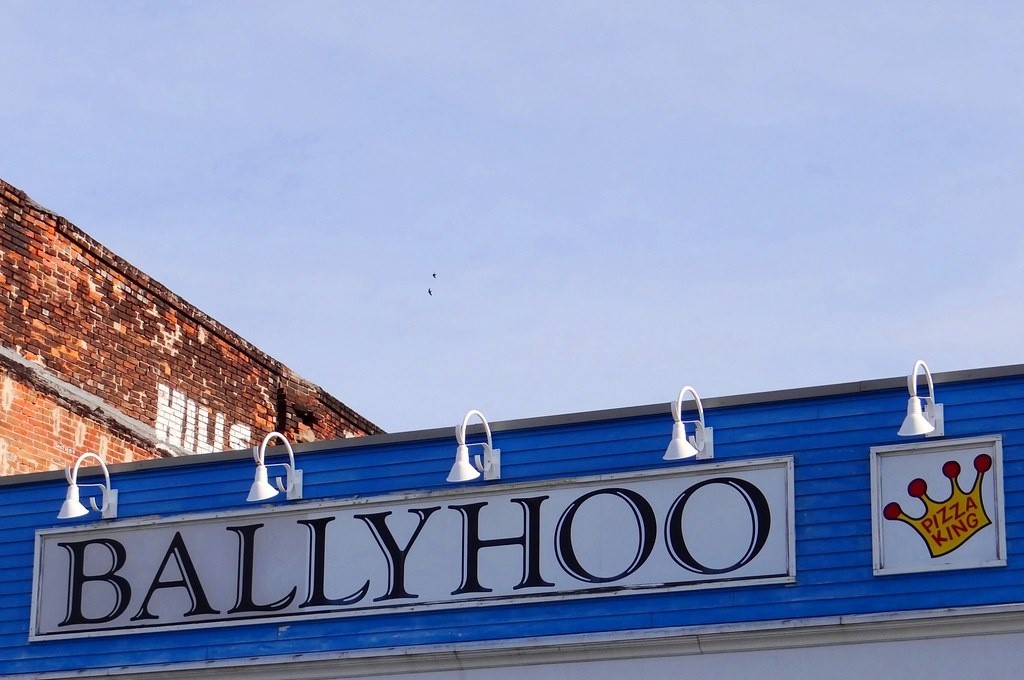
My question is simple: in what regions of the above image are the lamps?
[246,432,302,501]
[57,453,118,519]
[446,410,501,482]
[663,386,714,460]
[897,360,944,437]
